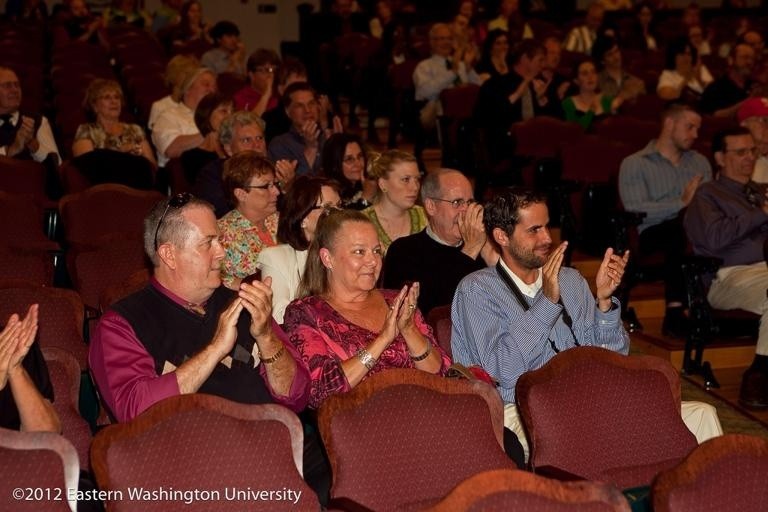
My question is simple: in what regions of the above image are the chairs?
[560,138,626,264]
[70,233,145,306]
[677,230,755,391]
[606,185,671,333]
[0,24,168,123]
[1,279,90,370]
[2,429,79,512]
[423,470,630,512]
[653,432,768,512]
[316,369,518,512]
[1,158,47,193]
[89,393,321,512]
[58,185,164,243]
[426,303,455,367]
[0,245,54,282]
[513,346,699,489]
[38,348,93,474]
[0,194,58,247]
[590,118,649,149]
[441,81,485,209]
[513,117,575,194]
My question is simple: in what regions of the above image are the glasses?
[341,151,366,163]
[152,190,196,253]
[237,180,281,191]
[311,200,348,213]
[428,195,476,209]
[718,144,759,159]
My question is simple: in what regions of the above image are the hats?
[736,96,768,125]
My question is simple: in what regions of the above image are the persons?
[1,302,106,512]
[283,204,525,470]
[685,125,767,407]
[0,1,767,337]
[451,185,724,464]
[85,191,331,508]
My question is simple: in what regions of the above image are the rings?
[410,304,413,309]
[390,305,395,310]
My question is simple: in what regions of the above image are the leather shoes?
[660,316,695,338]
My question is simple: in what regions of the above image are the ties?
[1,113,16,138]
[743,183,760,209]
[521,85,535,122]
[444,58,462,87]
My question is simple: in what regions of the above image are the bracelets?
[257,348,284,365]
[354,348,375,371]
[409,341,432,361]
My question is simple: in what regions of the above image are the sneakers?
[740,372,767,413]
